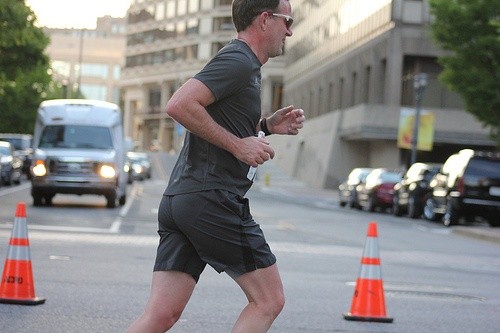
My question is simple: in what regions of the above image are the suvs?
[422,148,500,228]
[392,162,444,218]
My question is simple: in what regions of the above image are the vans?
[0,132,34,179]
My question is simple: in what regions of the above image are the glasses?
[267,12,293,30]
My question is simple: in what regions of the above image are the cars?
[0,140,23,187]
[338,167,376,208]
[353,167,401,212]
[127,152,151,184]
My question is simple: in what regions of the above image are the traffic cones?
[343,221,394,323]
[0,201,47,306]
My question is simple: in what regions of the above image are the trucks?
[28,98,127,209]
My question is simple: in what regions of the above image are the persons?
[122,0,305,333]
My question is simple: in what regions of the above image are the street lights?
[410,70,428,165]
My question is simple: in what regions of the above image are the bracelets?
[261,118,271,136]
